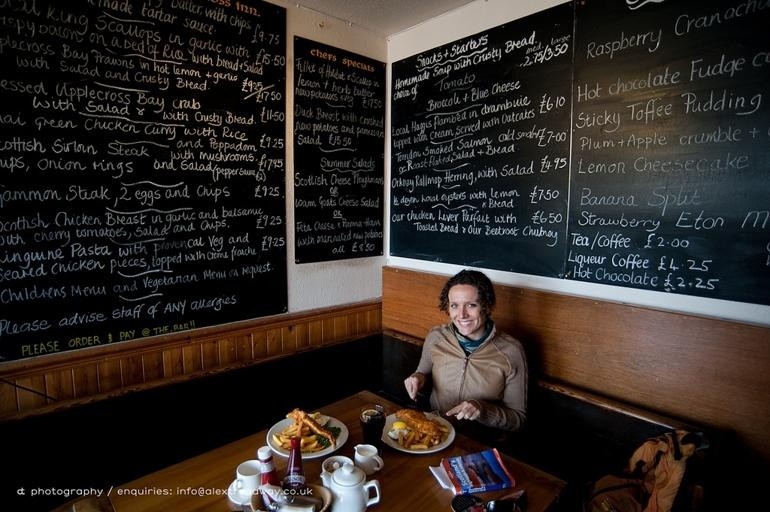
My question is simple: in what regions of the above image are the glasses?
[488,490,528,512]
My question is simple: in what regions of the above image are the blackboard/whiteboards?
[562,0,770,306]
[388,0,578,280]
[0,0,289,363]
[292,34,386,265]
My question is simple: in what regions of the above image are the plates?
[227,481,251,508]
[322,455,354,476]
[266,415,349,459]
[381,410,457,453]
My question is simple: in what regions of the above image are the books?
[440,448,515,496]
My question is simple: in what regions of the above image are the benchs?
[384,329,742,512]
[0,331,382,512]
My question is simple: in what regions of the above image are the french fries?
[273,424,324,452]
[397,430,432,450]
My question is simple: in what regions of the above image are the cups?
[353,443,383,475]
[360,403,387,442]
[233,460,262,499]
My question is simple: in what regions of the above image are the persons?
[404,269,529,445]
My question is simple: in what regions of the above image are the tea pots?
[320,462,382,512]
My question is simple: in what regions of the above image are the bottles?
[258,438,322,512]
